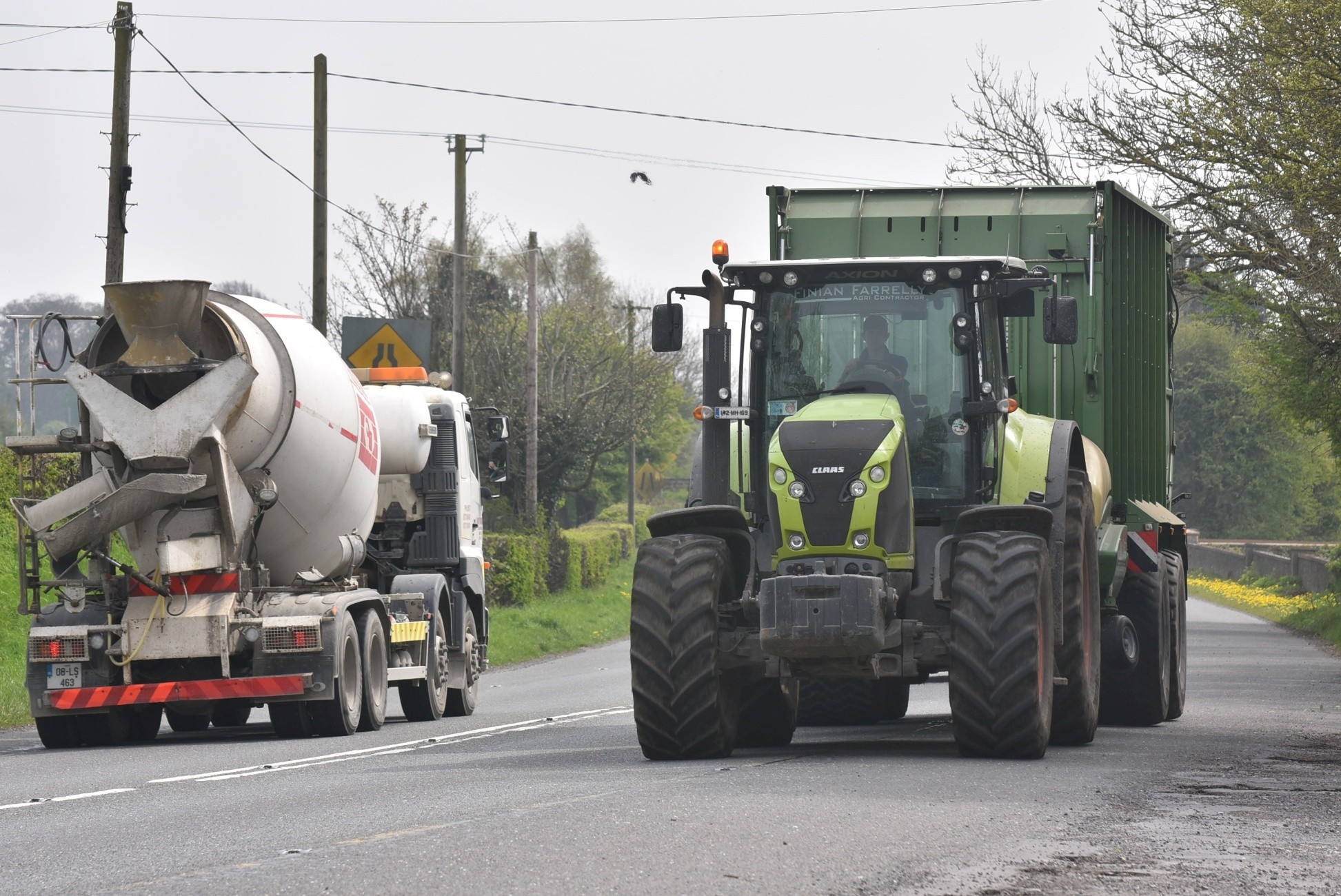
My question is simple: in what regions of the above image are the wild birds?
[630,172,651,185]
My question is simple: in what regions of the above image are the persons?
[838,314,907,416]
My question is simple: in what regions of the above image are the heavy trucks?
[4,279,512,749]
[630,179,1188,759]
